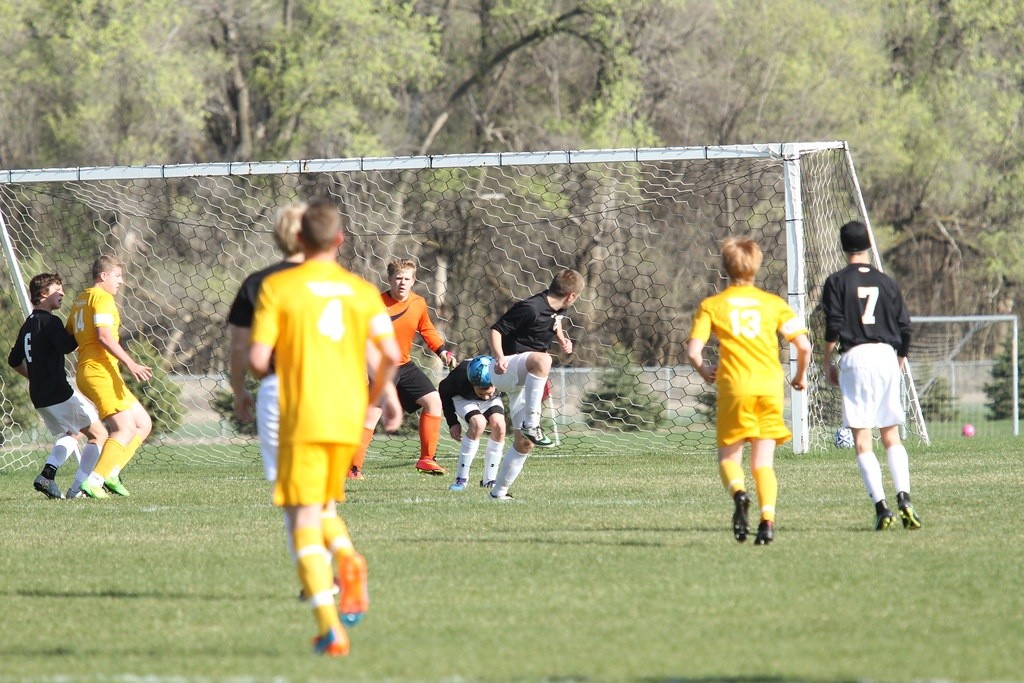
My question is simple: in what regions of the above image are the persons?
[439,355,506,490]
[687,237,810,546]
[487,270,585,500]
[227,201,341,600]
[67,255,152,499]
[347,259,456,480]
[823,222,922,530]
[249,202,399,654]
[8,273,108,498]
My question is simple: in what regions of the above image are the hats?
[841,221,872,251]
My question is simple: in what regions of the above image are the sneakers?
[315,626,350,658]
[67,488,90,500]
[449,479,467,490]
[338,556,366,629]
[81,481,110,499]
[520,421,555,448]
[488,491,515,502]
[877,509,893,530]
[733,493,753,542]
[899,501,922,529]
[480,479,496,488]
[347,467,362,479]
[756,519,773,544]
[105,478,131,496]
[415,457,446,476]
[33,475,65,499]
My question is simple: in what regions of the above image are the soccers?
[467,354,496,388]
[834,427,855,450]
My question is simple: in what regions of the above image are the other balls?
[962,424,976,436]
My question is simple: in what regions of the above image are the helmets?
[467,355,496,387]
